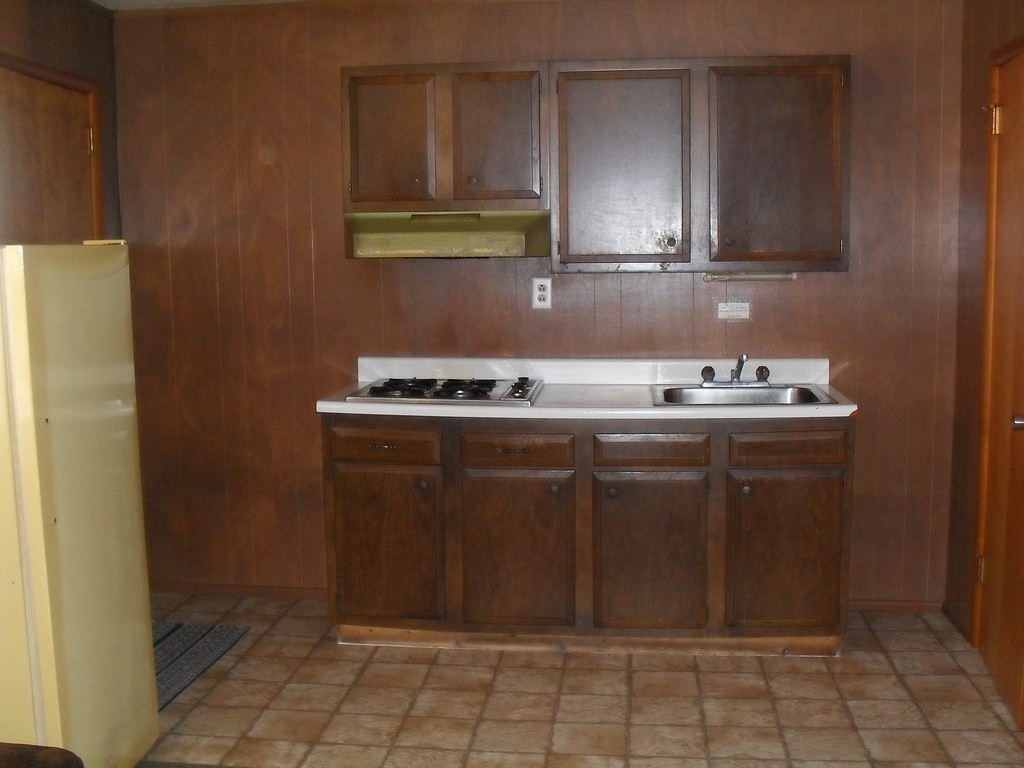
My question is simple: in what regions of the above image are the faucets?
[730,353,748,383]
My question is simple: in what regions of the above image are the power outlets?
[532,277,553,310]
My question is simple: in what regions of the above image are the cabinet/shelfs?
[323,411,856,658]
[342,56,852,272]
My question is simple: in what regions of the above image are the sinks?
[651,383,838,408]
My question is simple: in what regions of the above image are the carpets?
[151,620,252,711]
